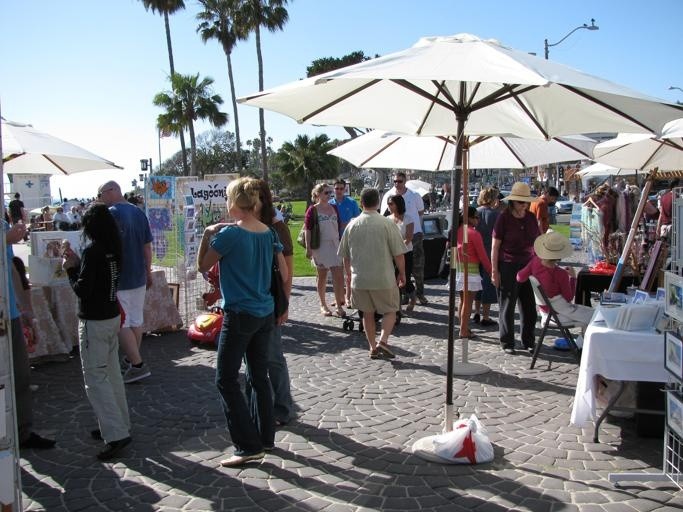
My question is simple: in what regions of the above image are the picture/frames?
[582,310,673,442]
[144,269,175,332]
[16,279,79,360]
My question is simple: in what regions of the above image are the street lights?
[271,261,289,318]
[438,247,451,279]
[297,222,320,250]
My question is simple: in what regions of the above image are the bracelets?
[202,234,211,239]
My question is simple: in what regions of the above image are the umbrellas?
[92,429,102,438]
[459,332,475,338]
[330,302,345,306]
[19,433,57,449]
[0,119,126,183]
[344,298,351,308]
[264,444,276,450]
[99,435,132,456]
[221,452,265,467]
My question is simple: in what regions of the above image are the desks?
[127,152,153,188]
[541,19,600,60]
[665,85,680,102]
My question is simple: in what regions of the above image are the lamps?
[526,274,581,370]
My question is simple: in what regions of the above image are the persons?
[97,179,153,386]
[274,203,284,223]
[281,205,290,225]
[3,207,56,451]
[530,186,560,235]
[304,173,417,359]
[195,173,279,468]
[244,177,294,426]
[453,181,538,355]
[1,190,145,232]
[53,199,140,460]
[516,231,594,347]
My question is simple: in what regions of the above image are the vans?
[335,307,346,317]
[319,305,332,316]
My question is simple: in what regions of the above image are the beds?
[0,119,126,183]
[576,117,682,179]
[325,123,603,368]
[231,32,682,437]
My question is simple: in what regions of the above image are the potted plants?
[662,270,682,441]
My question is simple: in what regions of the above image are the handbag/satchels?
[202,234,211,239]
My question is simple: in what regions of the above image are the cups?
[388,203,394,206]
[394,180,404,184]
[322,191,332,194]
[335,187,344,191]
[97,189,112,199]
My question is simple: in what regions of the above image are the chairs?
[481,318,497,326]
[417,295,428,304]
[123,364,151,383]
[119,359,131,372]
[473,314,480,321]
[376,341,395,358]
[395,311,403,325]
[500,347,514,354]
[406,297,416,313]
[370,348,381,358]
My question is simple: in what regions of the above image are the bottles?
[534,231,574,260]
[477,187,499,205]
[501,181,538,202]
